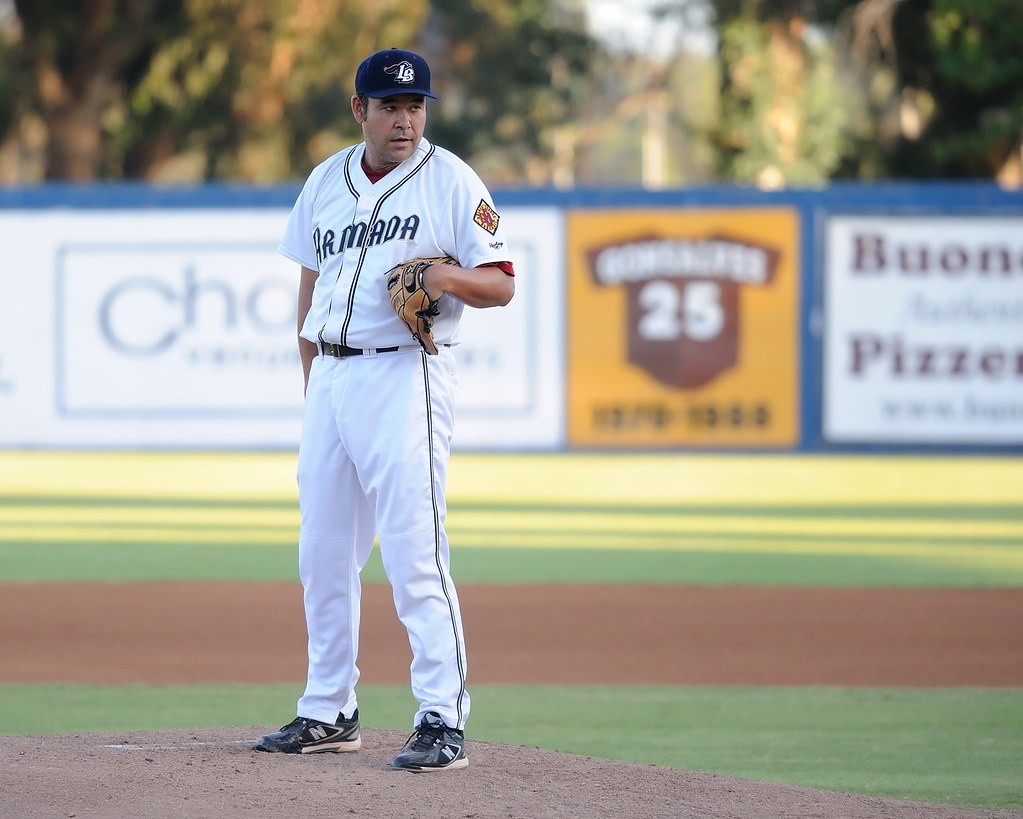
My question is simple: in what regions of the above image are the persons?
[256,44,516,773]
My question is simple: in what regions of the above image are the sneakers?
[254,708,361,754]
[391,711,469,771]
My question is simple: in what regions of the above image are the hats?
[355,47,438,99]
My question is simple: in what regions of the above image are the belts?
[321,342,450,359]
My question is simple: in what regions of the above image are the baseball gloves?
[382,255,462,356]
